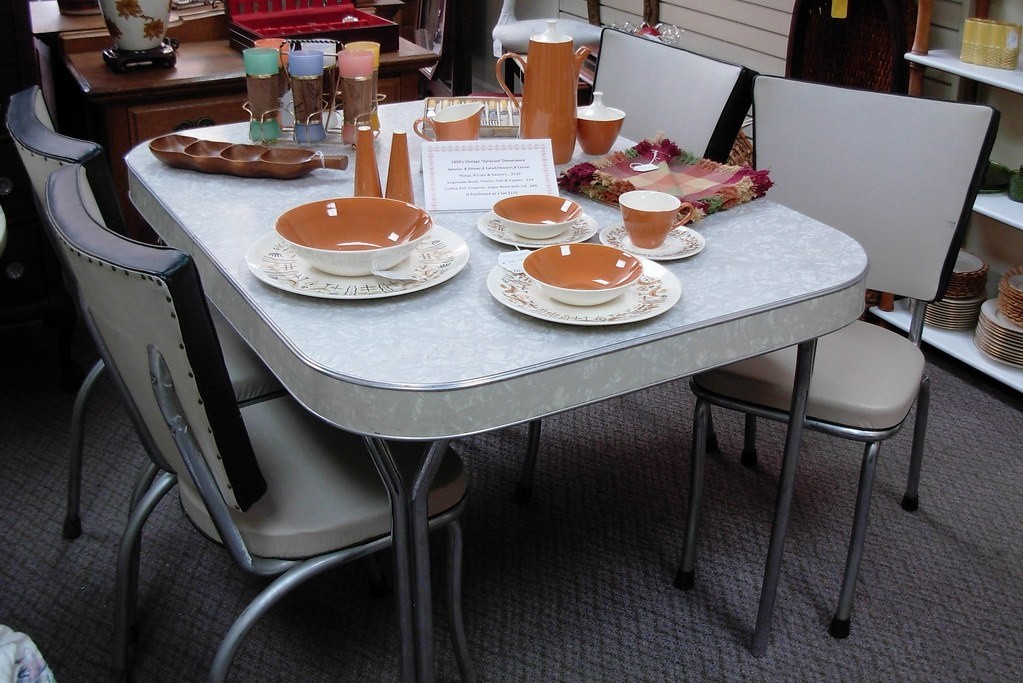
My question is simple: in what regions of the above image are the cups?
[1007,169,1023,203]
[619,190,695,250]
[577,92,627,156]
[244,38,380,144]
[413,102,486,142]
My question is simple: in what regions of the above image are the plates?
[599,222,705,260]
[977,160,1011,194]
[477,211,599,248]
[905,249,1023,369]
[486,254,682,326]
[245,224,470,299]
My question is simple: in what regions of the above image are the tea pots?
[495,20,592,165]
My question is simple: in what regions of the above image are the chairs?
[676,74,1002,643]
[44,161,483,683]
[3,85,288,623]
[517,26,760,507]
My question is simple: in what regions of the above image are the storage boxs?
[224,0,399,57]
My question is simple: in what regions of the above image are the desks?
[123,95,871,683]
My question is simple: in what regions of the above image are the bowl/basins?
[492,195,584,240]
[274,196,435,277]
[522,243,644,307]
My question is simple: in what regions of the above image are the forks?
[480,101,487,126]
[489,99,499,126]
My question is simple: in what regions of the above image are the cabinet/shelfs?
[61,35,440,247]
[865,0,1023,414]
